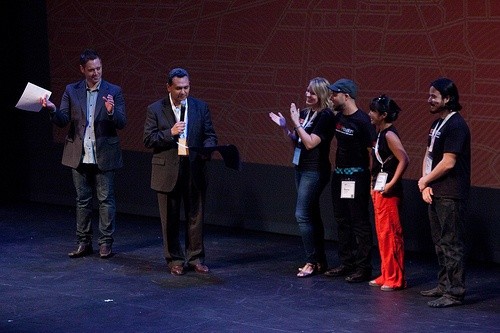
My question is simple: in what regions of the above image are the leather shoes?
[170,265,185,275]
[189,263,209,273]
[68,245,92,258]
[99,245,112,258]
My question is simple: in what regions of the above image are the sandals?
[296,262,320,277]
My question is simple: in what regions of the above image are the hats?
[326,78,357,99]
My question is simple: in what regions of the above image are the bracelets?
[294,125,302,130]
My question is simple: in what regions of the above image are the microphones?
[180,100,186,121]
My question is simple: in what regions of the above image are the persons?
[417,78,472,307]
[143,68,218,276]
[39,50,128,257]
[326,79,375,282]
[269,78,336,278]
[368,96,410,290]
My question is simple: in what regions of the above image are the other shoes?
[323,264,352,277]
[427,297,459,307]
[344,271,371,281]
[369,280,379,286]
[380,284,393,292]
[419,286,442,297]
